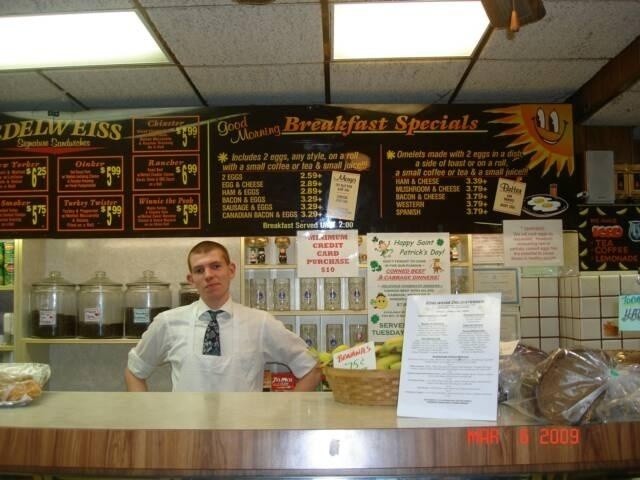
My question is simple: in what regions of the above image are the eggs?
[527,197,561,212]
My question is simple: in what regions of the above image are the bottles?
[32,272,200,341]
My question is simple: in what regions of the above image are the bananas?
[318,335,403,371]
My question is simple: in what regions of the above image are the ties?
[202,310,225,356]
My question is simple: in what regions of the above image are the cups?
[249,278,365,354]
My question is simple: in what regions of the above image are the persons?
[121,240,327,391]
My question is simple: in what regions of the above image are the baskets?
[322,365,402,406]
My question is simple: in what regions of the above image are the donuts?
[592,225,623,238]
